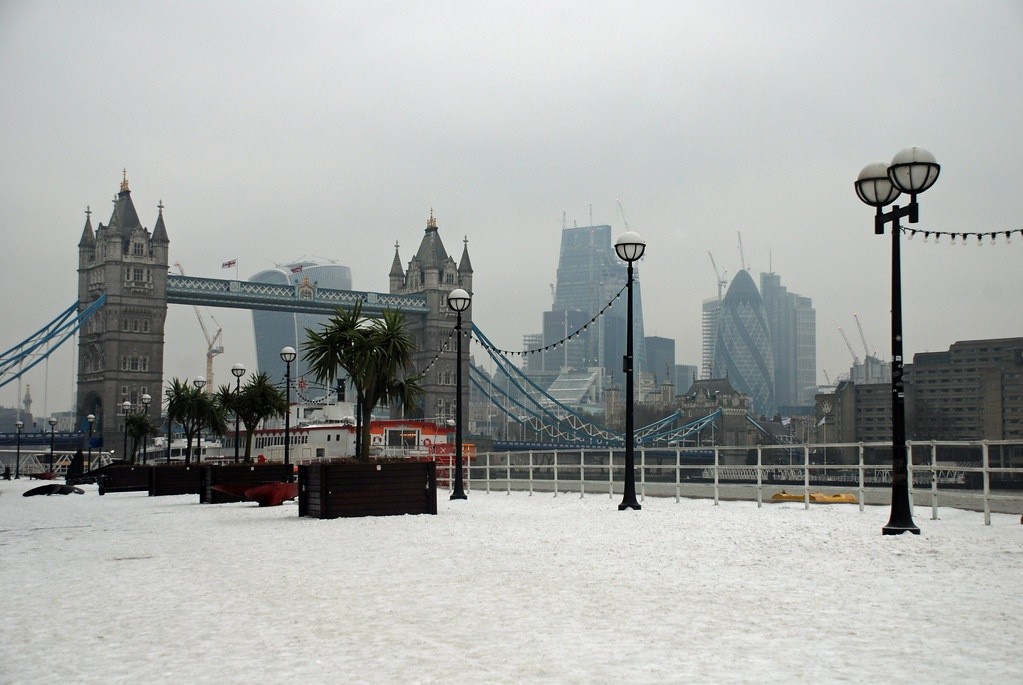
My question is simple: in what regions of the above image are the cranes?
[613,198,631,233]
[733,229,750,273]
[821,313,879,386]
[174,262,226,396]
[708,249,728,300]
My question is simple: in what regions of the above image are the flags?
[290,265,303,273]
[221,259,237,269]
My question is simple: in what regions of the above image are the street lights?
[192,375,207,463]
[16,420,24,478]
[87,414,96,472]
[122,400,131,461]
[281,346,297,467]
[614,232,647,510]
[231,363,246,463]
[444,289,474,497]
[859,144,940,534]
[142,394,152,466]
[48,418,56,473]
[166,387,176,463]
